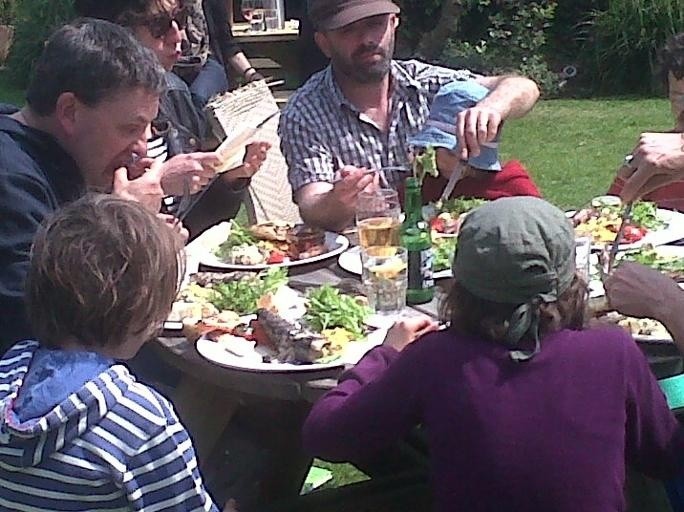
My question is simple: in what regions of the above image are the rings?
[623,155,634,166]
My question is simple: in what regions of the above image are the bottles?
[399,176,436,306]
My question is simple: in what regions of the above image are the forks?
[329,159,420,183]
[173,175,192,224]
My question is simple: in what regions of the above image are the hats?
[306,0,401,34]
[404,77,503,171]
[450,194,578,305]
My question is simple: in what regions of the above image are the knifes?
[436,157,470,202]
[606,202,633,269]
[177,171,221,224]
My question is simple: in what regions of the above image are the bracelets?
[244,68,256,78]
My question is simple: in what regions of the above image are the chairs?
[201,79,303,225]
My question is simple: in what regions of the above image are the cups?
[355,188,399,227]
[359,244,408,312]
[241,0,285,32]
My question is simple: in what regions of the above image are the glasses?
[129,6,191,40]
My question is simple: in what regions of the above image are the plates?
[196,313,367,373]
[574,244,684,300]
[199,227,350,267]
[563,202,683,253]
[336,244,408,277]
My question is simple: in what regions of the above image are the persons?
[0,191,236,512]
[0,17,189,358]
[605,34,684,215]
[371,82,542,206]
[300,195,684,512]
[276,0,541,233]
[602,133,684,357]
[179,0,264,143]
[73,0,272,243]
